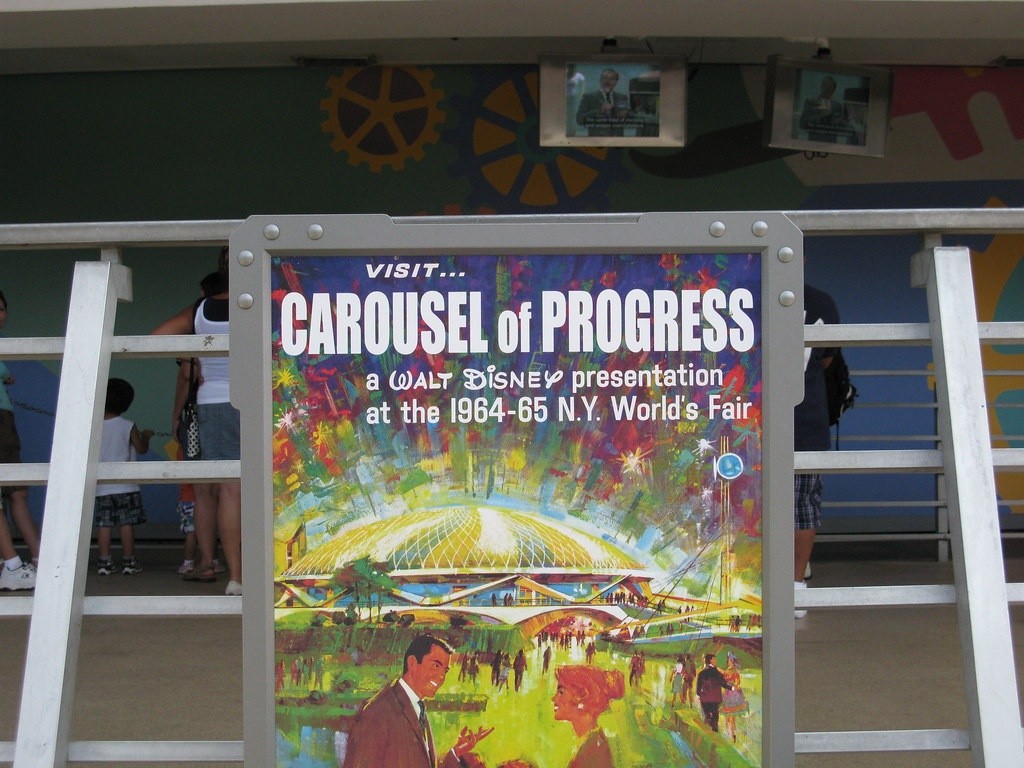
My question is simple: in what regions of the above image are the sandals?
[184,561,217,582]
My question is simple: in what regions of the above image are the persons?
[794,285,831,619]
[150,246,240,596]
[800,77,849,144]
[93,378,154,575]
[566,61,630,137]
[0,291,40,591]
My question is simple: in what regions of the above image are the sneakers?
[98,559,119,575]
[0,561,37,590]
[122,559,143,574]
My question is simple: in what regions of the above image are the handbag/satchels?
[183,404,201,460]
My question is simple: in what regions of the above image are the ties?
[606,92,611,104]
[825,99,829,111]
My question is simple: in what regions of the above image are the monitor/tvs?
[538,51,689,147]
[760,54,891,159]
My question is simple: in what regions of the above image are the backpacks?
[817,349,856,428]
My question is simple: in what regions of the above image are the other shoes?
[214,562,225,573]
[803,562,812,578]
[178,562,195,573]
[794,583,807,619]
[225,580,242,595]
